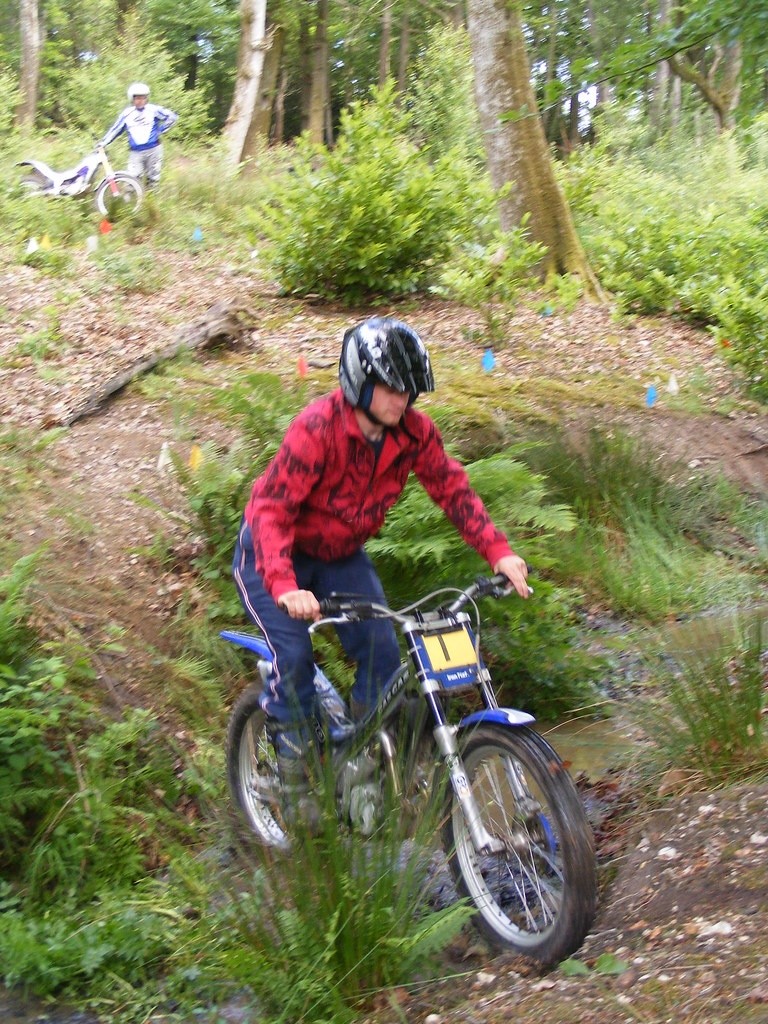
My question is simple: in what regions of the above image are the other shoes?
[349,694,375,722]
[277,756,322,827]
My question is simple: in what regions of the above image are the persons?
[232,318,530,830]
[93,82,180,188]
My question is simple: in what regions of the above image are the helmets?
[338,317,434,408]
[127,83,151,104]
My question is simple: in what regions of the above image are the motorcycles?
[12,130,145,225]
[219,572,600,969]
